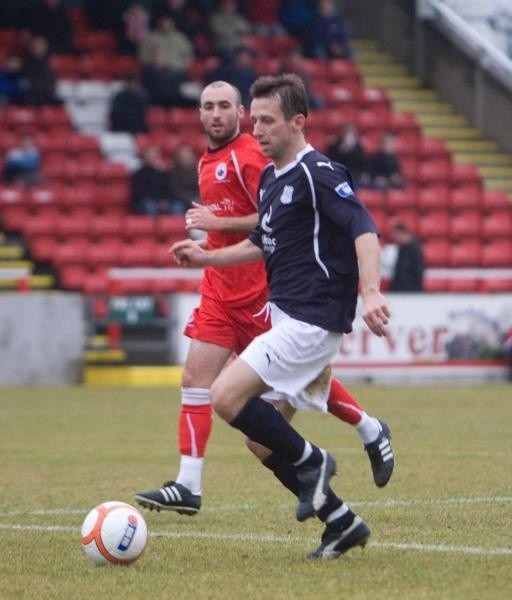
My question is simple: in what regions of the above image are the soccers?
[81,500,149,567]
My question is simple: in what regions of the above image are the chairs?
[0,3,512,292]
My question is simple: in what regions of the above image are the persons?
[132,79,398,517]
[361,132,406,189]
[386,220,425,291]
[169,144,203,202]
[1,133,44,186]
[324,123,368,186]
[166,72,396,561]
[128,143,187,220]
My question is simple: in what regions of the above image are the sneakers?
[294,448,337,522]
[133,481,202,515]
[307,515,372,560]
[364,416,395,488]
